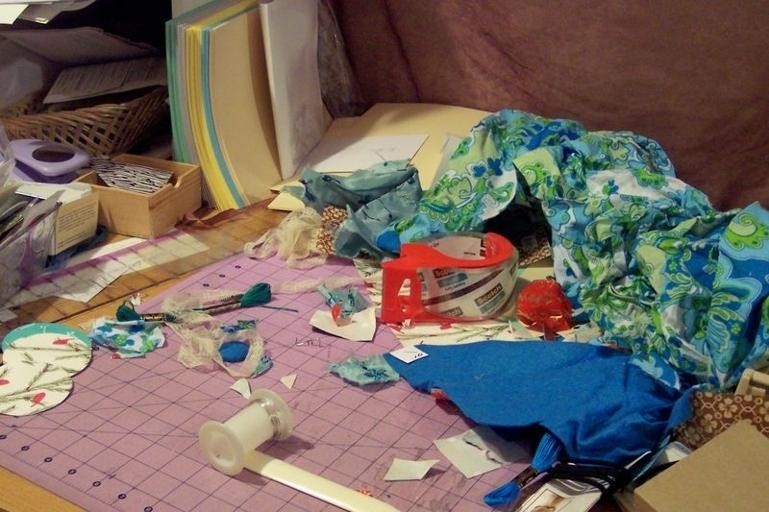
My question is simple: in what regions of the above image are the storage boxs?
[2,149,203,307]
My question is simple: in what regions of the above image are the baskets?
[0,79,168,159]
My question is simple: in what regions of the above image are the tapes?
[417,231,518,320]
